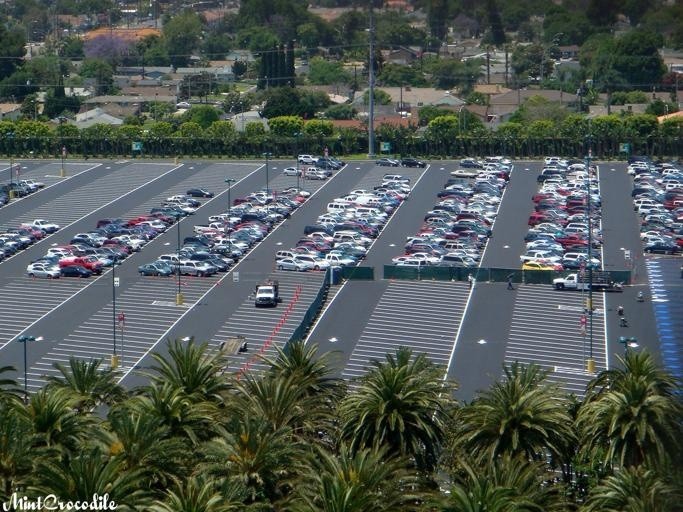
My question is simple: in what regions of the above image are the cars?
[0,179,274,281]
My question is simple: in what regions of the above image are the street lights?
[224,177,235,255]
[56,116,69,179]
[4,130,18,189]
[16,331,45,409]
[617,336,640,371]
[259,150,273,193]
[578,263,600,375]
[103,257,122,368]
[293,132,303,195]
[171,206,187,308]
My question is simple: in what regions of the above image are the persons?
[507,276,515,290]
[468,273,473,288]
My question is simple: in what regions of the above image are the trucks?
[550,272,613,292]
[252,275,284,308]
